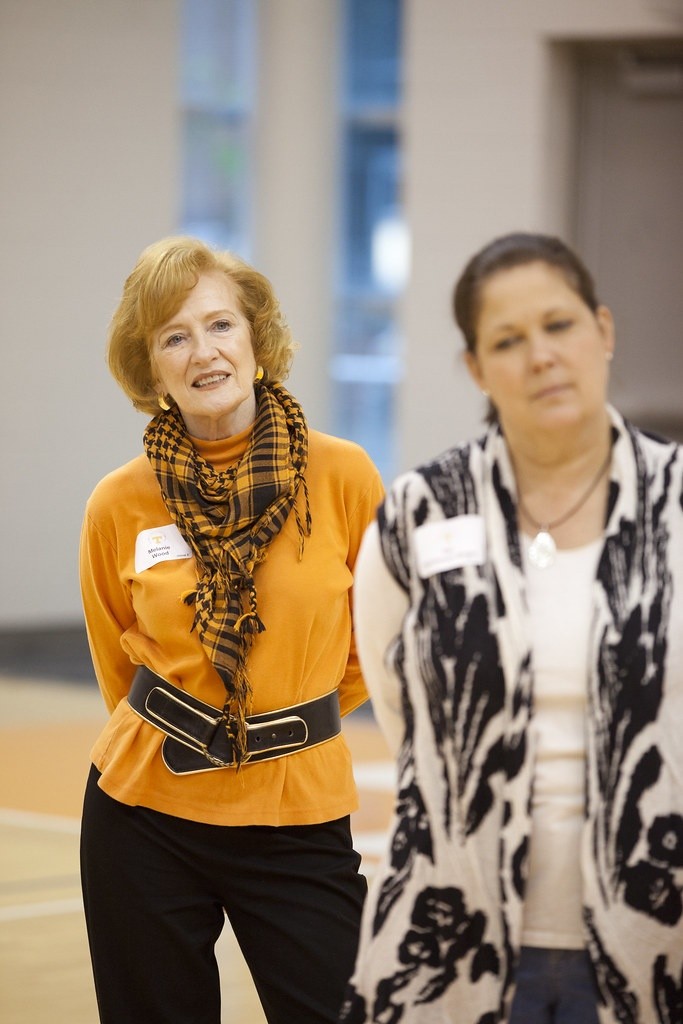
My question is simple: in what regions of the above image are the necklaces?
[512,437,615,569]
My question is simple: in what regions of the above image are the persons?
[335,229,683,1023]
[75,229,389,1024]
